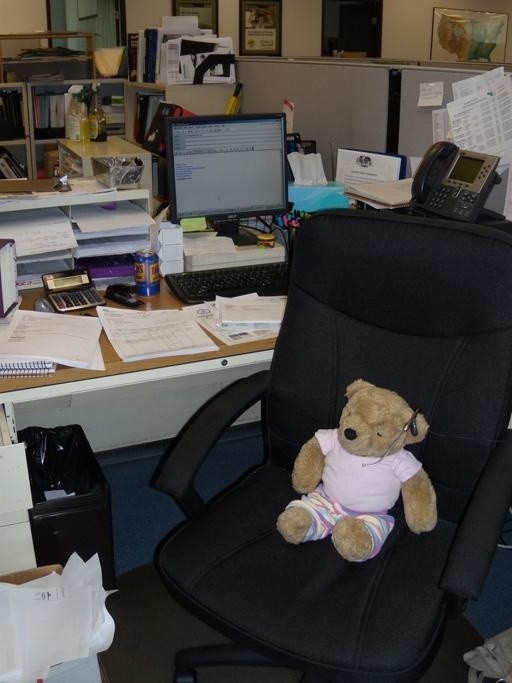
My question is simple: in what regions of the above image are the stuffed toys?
[275,379,439,562]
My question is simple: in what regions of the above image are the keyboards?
[163,261,290,303]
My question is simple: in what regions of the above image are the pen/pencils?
[275,209,311,228]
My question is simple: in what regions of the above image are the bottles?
[84,85,89,104]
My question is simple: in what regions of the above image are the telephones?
[408,140,503,223]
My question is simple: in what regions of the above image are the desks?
[0,276,279,444]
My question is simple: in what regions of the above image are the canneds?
[135,250,160,298]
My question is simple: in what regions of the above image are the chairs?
[150,208,512,683]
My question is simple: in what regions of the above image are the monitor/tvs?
[164,112,304,247]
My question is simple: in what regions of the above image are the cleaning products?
[64,80,109,144]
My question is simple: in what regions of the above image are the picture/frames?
[239,0,282,57]
[172,0,218,39]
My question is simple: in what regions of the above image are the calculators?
[40,266,107,314]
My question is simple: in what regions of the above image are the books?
[343,177,414,210]
[0,292,288,379]
[0,89,65,179]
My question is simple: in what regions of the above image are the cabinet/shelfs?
[0,32,96,82]
[0,82,33,182]
[27,78,127,180]
